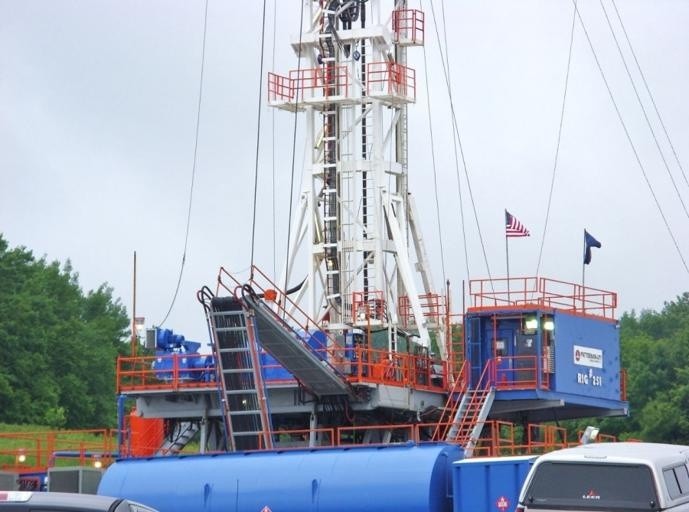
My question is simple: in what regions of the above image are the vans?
[515,441,688,511]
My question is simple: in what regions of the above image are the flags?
[505,211,530,237]
[583,231,601,263]
